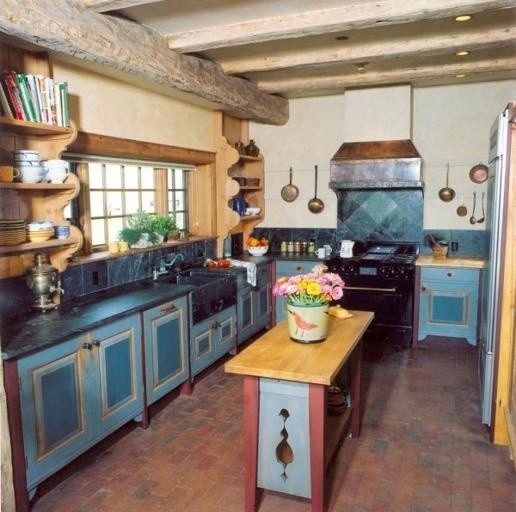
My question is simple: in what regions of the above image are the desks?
[224,310,374,512]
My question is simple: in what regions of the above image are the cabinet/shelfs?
[216,136,265,240]
[236,262,273,346]
[273,260,324,329]
[0,118,84,279]
[413,265,484,347]
[3,311,145,512]
[142,293,189,427]
[189,304,236,393]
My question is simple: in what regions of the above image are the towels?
[226,258,256,287]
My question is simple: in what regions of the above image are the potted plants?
[153,216,178,242]
[116,208,164,249]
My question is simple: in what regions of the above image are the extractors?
[329,140,424,189]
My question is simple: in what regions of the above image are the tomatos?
[209,261,231,268]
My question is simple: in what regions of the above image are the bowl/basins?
[12,159,41,167]
[18,167,43,183]
[246,179,260,186]
[232,177,246,185]
[247,245,268,256]
[246,207,261,215]
[13,150,39,159]
[42,159,68,185]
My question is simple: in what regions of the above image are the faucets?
[158,254,184,273]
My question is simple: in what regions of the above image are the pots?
[281,167,299,202]
[308,165,324,213]
[470,160,495,184]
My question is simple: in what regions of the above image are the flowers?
[272,264,346,306]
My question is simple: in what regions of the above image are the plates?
[27,223,54,242]
[53,225,70,239]
[0,219,26,246]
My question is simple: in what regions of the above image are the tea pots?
[329,304,353,319]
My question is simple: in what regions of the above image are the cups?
[0,166,20,183]
[118,240,129,252]
[323,245,332,255]
[109,243,121,253]
[315,248,326,259]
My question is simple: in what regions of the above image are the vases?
[287,304,330,343]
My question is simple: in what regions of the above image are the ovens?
[329,280,415,349]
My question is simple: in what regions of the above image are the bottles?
[294,242,301,253]
[288,242,294,253]
[281,242,288,253]
[308,242,315,254]
[301,242,308,253]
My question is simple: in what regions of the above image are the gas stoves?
[323,240,420,281]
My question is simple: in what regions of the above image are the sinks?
[155,271,229,286]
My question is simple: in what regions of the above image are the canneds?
[280,241,315,253]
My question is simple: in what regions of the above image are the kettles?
[336,240,355,259]
